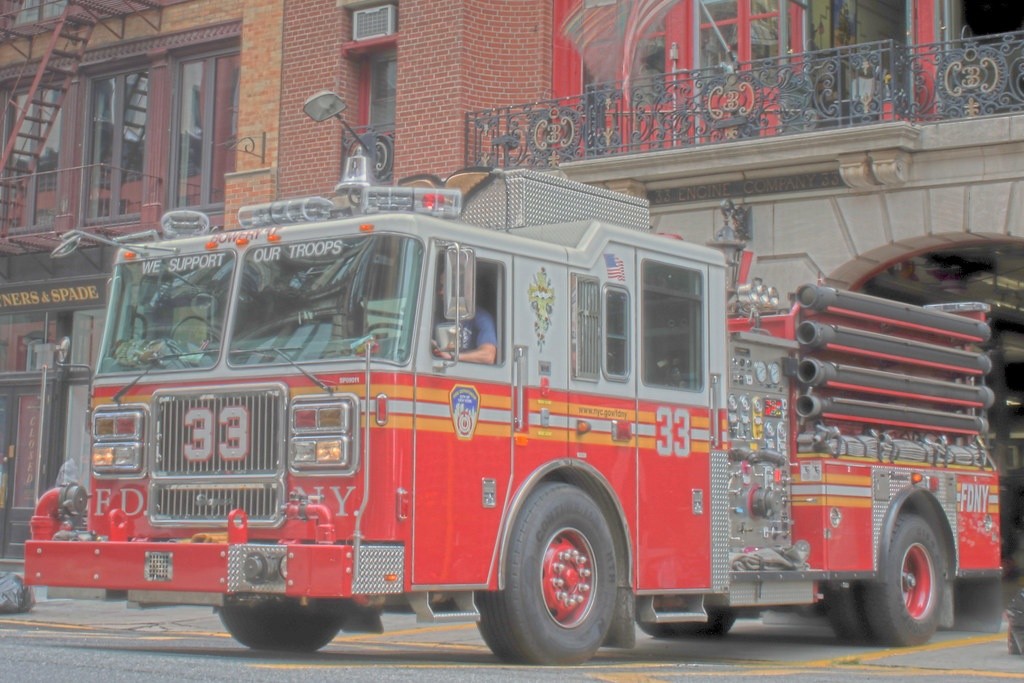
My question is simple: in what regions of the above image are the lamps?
[302,89,378,168]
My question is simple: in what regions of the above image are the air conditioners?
[352,4,399,42]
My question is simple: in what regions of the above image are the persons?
[432,266,497,364]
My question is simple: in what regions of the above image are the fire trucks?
[20,162,1003,667]
